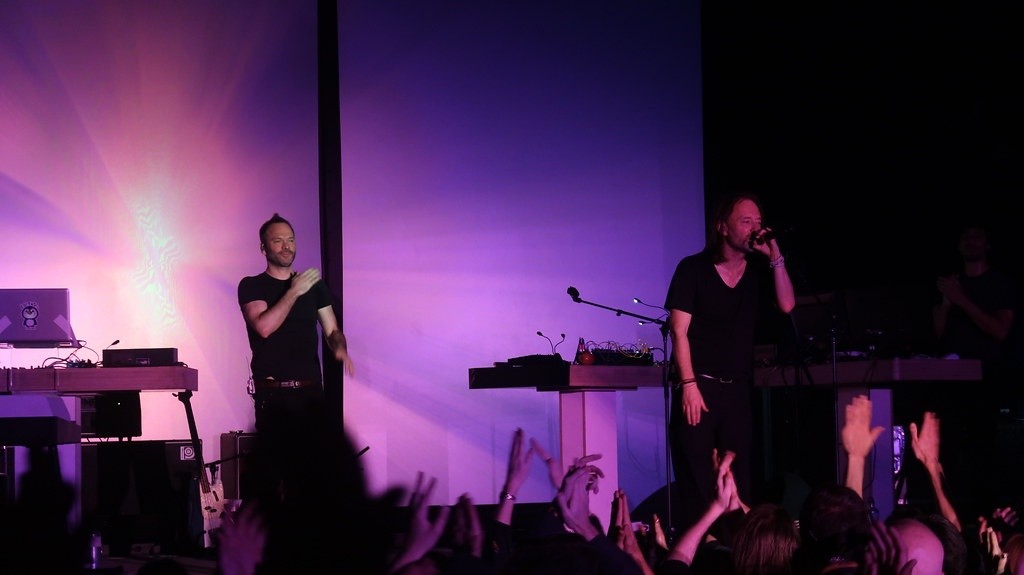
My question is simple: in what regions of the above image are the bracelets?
[770,254,785,268]
[501,491,516,500]
[675,377,697,389]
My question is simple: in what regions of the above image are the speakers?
[630,481,687,528]
[81,390,142,438]
[80,440,203,560]
[220,432,257,511]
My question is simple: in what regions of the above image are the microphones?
[756,232,778,245]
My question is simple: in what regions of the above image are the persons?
[135,411,1024,575]
[663,193,797,517]
[934,225,1016,397]
[237,211,348,499]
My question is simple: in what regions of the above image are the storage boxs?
[82,439,204,555]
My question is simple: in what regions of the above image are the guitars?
[177,390,224,550]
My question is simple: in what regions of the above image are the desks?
[468,368,678,537]
[0,366,197,567]
[751,358,983,526]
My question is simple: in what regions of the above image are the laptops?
[0,289,82,349]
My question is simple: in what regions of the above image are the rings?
[655,518,659,523]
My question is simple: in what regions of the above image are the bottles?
[572,338,586,366]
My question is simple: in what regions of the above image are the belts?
[696,370,744,386]
[256,379,317,389]
